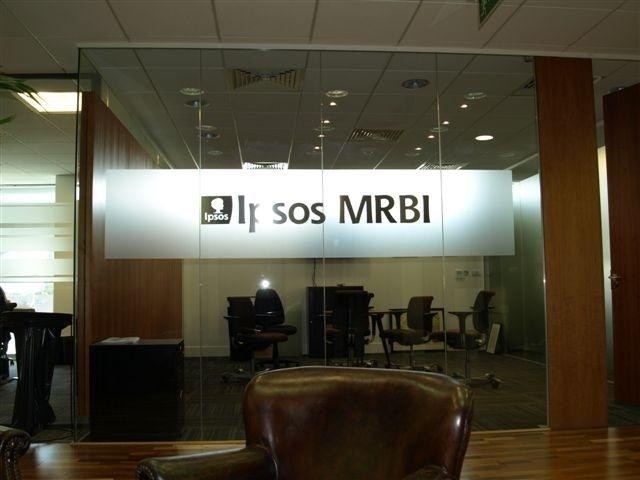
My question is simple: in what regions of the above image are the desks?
[1,310,74,437]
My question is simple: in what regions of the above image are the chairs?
[217,282,510,392]
[0,425,34,478]
[133,370,475,480]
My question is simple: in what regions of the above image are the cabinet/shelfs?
[89,335,187,442]
